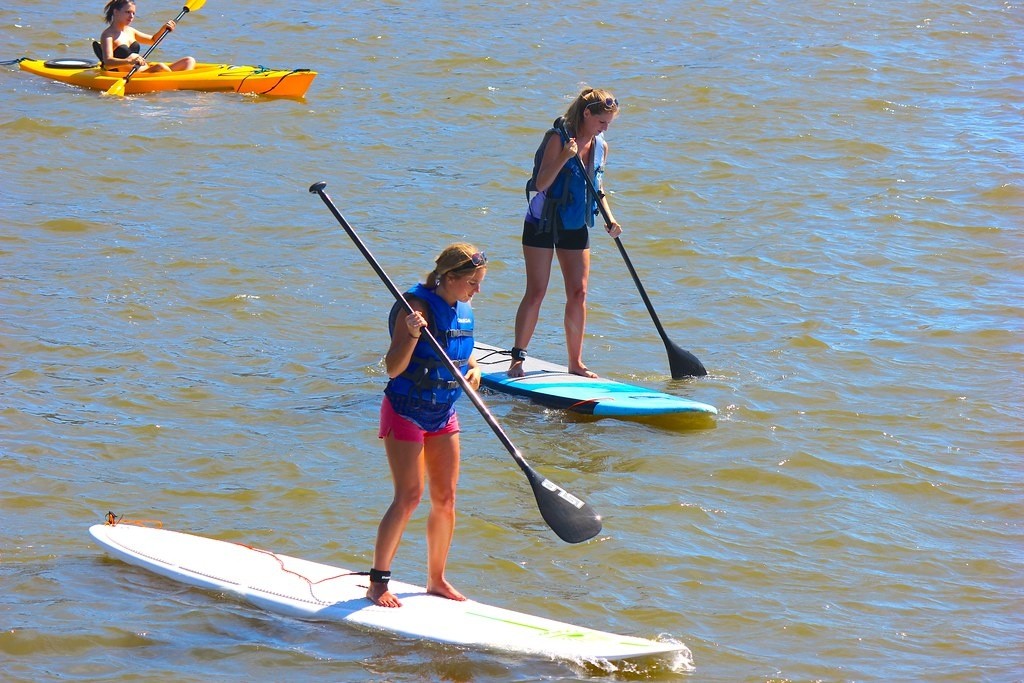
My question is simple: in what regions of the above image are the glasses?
[445,252,488,275]
[586,98,619,109]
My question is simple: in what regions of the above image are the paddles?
[305,175,611,549]
[552,112,716,385]
[100,0,209,101]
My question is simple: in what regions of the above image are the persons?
[367,242,488,608]
[100,0,195,72]
[508,87,622,379]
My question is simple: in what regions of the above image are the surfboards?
[468,339,717,420]
[87,520,678,663]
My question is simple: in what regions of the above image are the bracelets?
[408,333,419,338]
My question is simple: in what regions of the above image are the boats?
[15,55,318,100]
